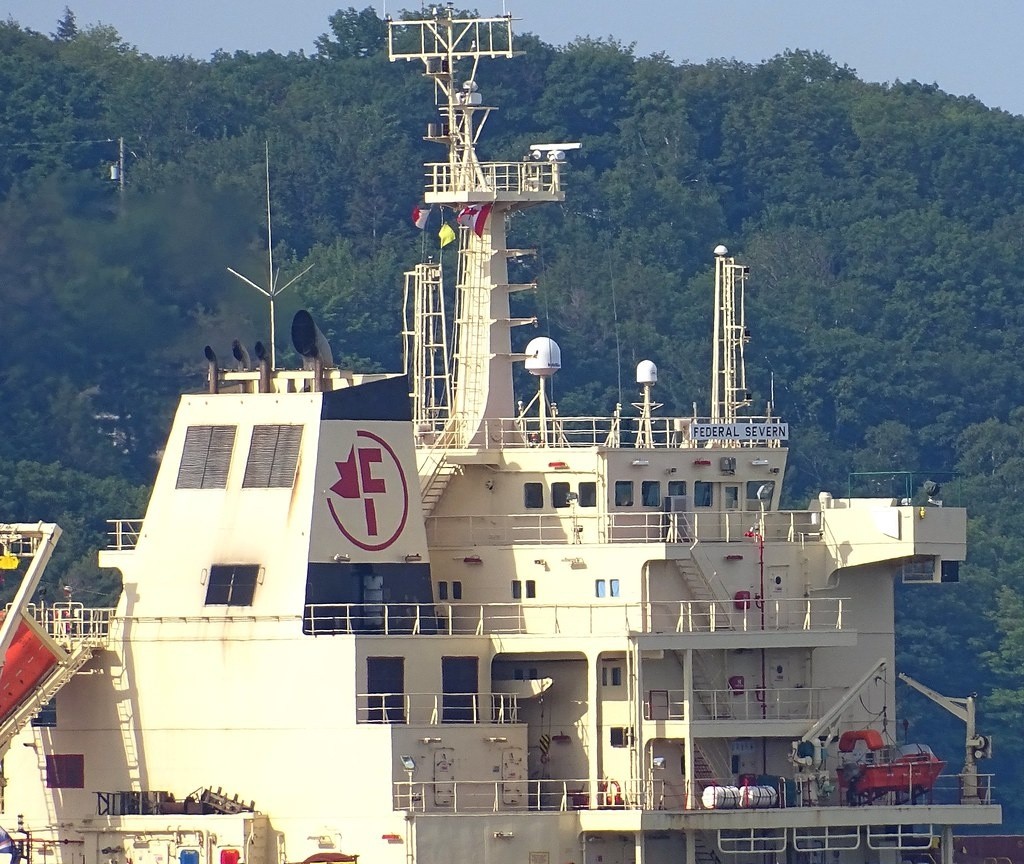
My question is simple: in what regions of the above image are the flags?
[439,222,456,248]
[412,206,431,229]
[457,203,494,238]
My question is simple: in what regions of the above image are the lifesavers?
[602,781,621,807]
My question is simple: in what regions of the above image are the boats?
[0,7,1006,864]
[834,751,948,794]
